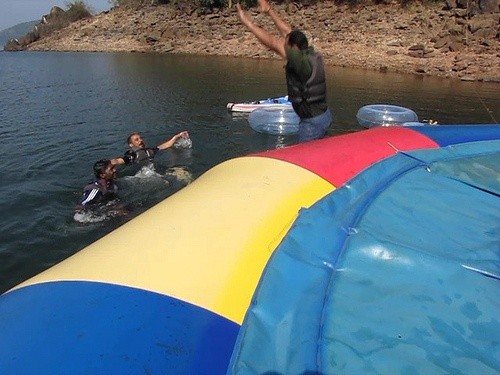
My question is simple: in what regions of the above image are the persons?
[76,159,119,209]
[111,131,189,176]
[236,0,334,144]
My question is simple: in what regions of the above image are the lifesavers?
[248,104,301,135]
[356,104,419,129]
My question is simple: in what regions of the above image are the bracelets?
[266,6,271,14]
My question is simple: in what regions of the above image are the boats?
[226,94,293,112]
